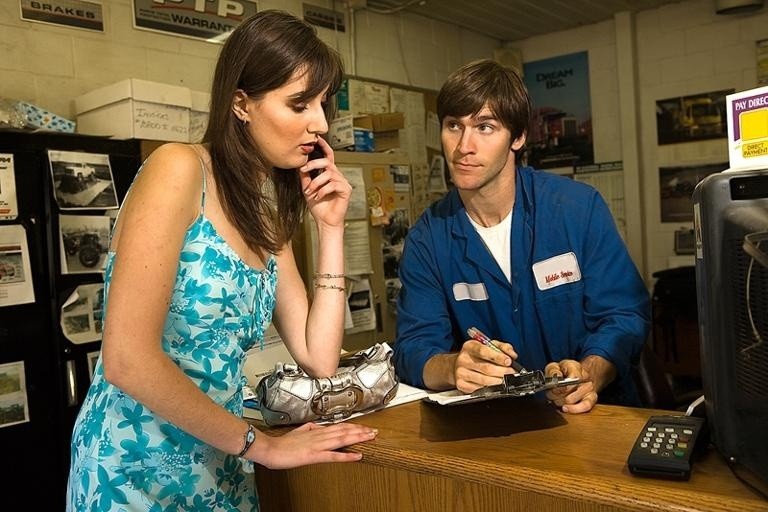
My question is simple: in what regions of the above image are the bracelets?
[312,282,347,292]
[312,270,345,281]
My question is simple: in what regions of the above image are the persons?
[65,6,382,511]
[384,57,651,420]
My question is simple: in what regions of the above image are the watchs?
[234,418,256,459]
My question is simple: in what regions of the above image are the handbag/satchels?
[252,337,400,428]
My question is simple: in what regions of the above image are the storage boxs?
[75,78,213,143]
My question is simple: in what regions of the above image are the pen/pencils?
[467,326,529,374]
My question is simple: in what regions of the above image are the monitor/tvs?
[692,169,768,495]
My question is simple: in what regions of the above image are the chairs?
[630,265,704,410]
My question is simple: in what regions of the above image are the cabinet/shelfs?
[0,129,415,512]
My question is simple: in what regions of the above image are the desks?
[243,388,768,512]
